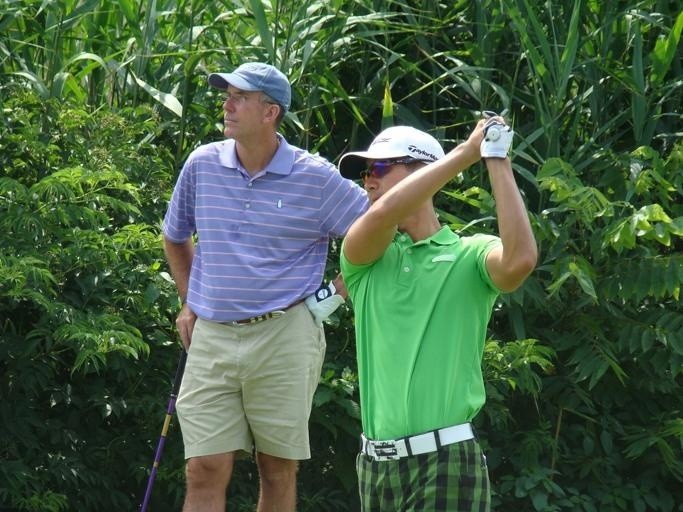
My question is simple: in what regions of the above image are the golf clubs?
[487,107,510,141]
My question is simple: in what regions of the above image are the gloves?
[480,110,512,159]
[304,280,345,324]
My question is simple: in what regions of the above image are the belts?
[221,298,304,327]
[360,423,475,462]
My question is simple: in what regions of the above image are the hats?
[338,126,446,180]
[207,62,291,111]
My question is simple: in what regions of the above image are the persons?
[338,110,537,512]
[161,62,368,511]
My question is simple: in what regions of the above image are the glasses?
[359,158,432,183]
[217,91,248,106]
